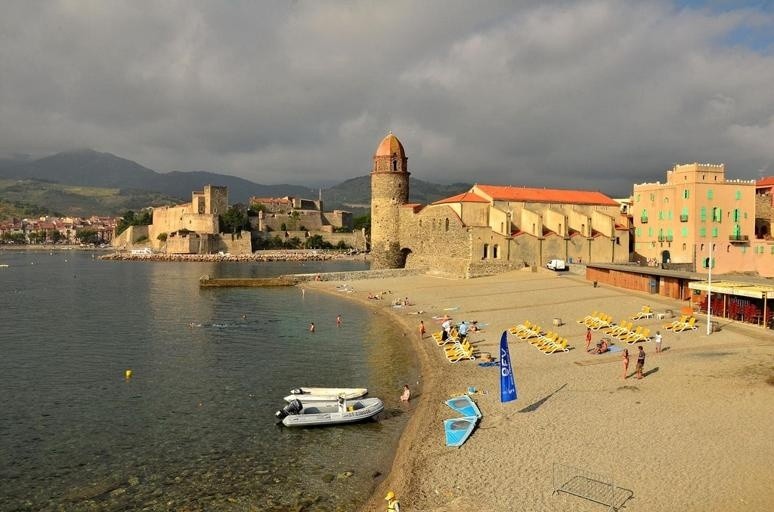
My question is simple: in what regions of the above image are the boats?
[275,397,383,427]
[283,387,367,403]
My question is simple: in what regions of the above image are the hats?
[385,491,395,501]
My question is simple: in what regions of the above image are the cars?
[546,259,565,271]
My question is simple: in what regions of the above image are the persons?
[655,331,662,353]
[400,385,411,401]
[592,339,607,354]
[636,346,645,379]
[441,315,477,344]
[383,492,400,512]
[648,257,658,267]
[392,297,409,308]
[309,323,315,333]
[368,288,391,300]
[419,320,424,339]
[336,315,342,328]
[585,328,592,352]
[622,348,629,381]
[315,275,320,281]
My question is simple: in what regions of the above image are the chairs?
[581,304,699,345]
[510,321,571,355]
[432,325,475,364]
